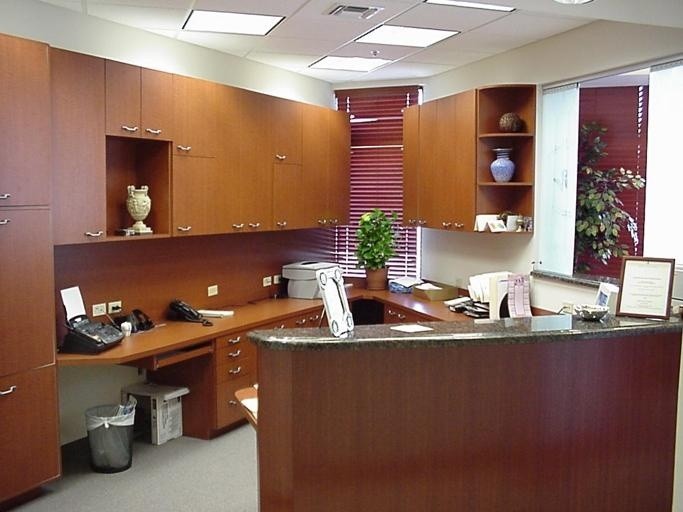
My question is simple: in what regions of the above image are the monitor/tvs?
[593,282,620,314]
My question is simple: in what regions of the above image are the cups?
[121,322,131,337]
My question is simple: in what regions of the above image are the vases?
[487,145,517,183]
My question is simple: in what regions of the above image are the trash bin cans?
[85,404,136,473]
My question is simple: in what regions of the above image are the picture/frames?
[613,254,676,322]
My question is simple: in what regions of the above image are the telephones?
[168,300,203,321]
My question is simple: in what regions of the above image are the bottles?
[125,185,151,231]
[498,112,522,133]
[488,148,515,182]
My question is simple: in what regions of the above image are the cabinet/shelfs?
[401,99,437,230]
[412,298,474,326]
[372,289,418,326]
[434,87,478,234]
[473,81,537,235]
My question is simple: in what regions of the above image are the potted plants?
[353,206,401,290]
